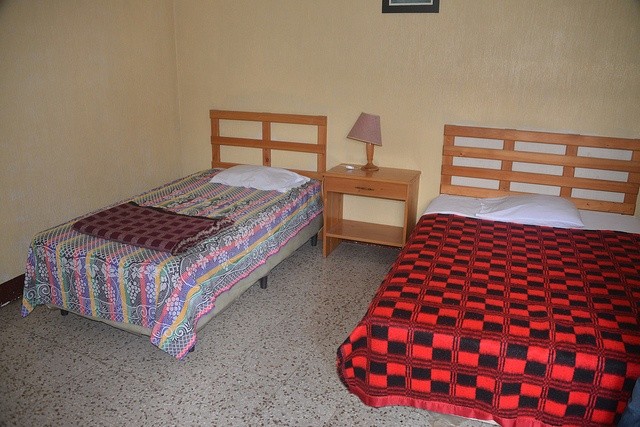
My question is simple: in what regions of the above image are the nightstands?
[322,162,421,258]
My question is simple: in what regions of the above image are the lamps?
[346,111,384,172]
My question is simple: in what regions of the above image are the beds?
[20,109,327,360]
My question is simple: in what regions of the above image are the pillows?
[210,164,311,193]
[335,124,640,427]
[476,193,585,229]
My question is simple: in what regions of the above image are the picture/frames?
[382,0,440,14]
